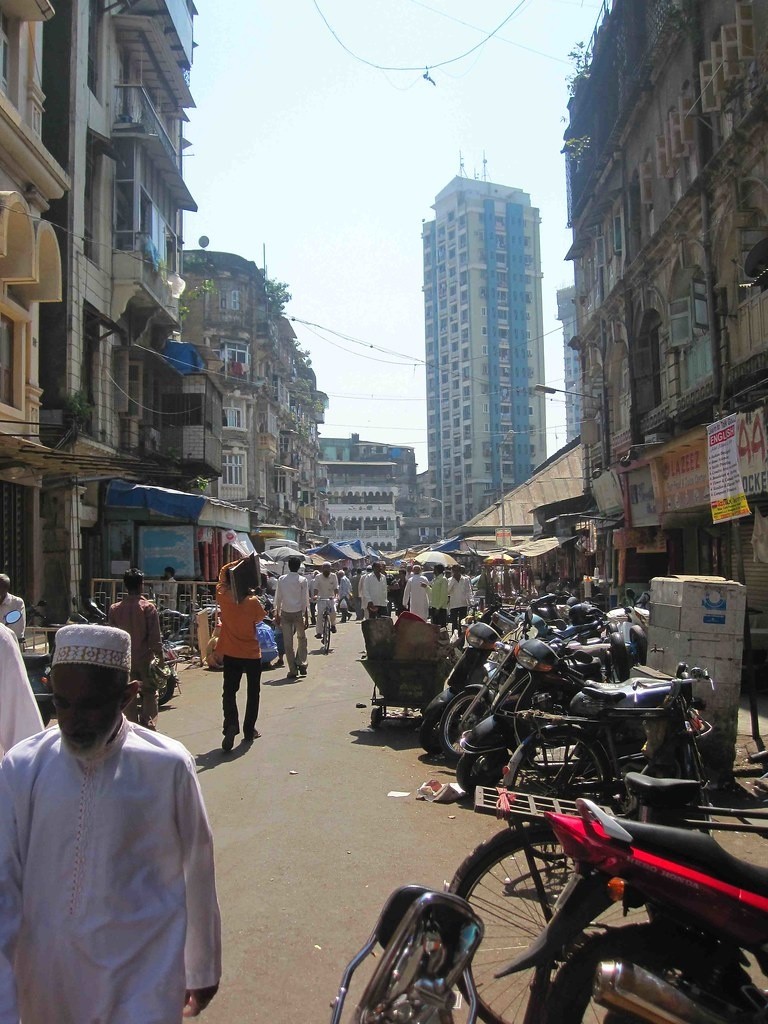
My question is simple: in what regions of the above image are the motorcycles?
[21,597,213,729]
[324,599,768,1024]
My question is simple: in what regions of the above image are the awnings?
[101,481,250,532]
[503,537,577,557]
[432,535,463,553]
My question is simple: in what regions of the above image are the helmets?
[569,603,591,624]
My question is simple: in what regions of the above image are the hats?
[51,623,131,672]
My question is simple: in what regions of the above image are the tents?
[304,534,390,566]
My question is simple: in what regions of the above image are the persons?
[363,562,389,619]
[0,624,222,1024]
[108,568,165,730]
[216,556,268,751]
[160,567,178,617]
[0,623,44,761]
[302,565,372,625]
[461,564,535,592]
[402,565,430,623]
[312,561,339,639]
[420,563,448,629]
[0,573,26,639]
[273,558,310,679]
[447,564,474,635]
[379,561,414,617]
[255,573,286,665]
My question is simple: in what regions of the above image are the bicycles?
[315,607,335,653]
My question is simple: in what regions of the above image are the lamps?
[621,442,664,466]
[592,465,620,481]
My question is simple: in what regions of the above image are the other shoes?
[245,732,261,740]
[222,725,237,751]
[287,672,296,678]
[340,621,346,623]
[274,660,283,667]
[300,666,307,675]
[316,634,320,639]
[331,626,336,633]
[348,614,352,620]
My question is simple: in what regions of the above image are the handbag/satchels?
[340,599,347,609]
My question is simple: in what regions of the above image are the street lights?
[303,498,329,531]
[532,384,619,587]
[423,496,446,540]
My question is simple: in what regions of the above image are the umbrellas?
[414,551,459,568]
[484,553,514,564]
[260,543,307,575]
